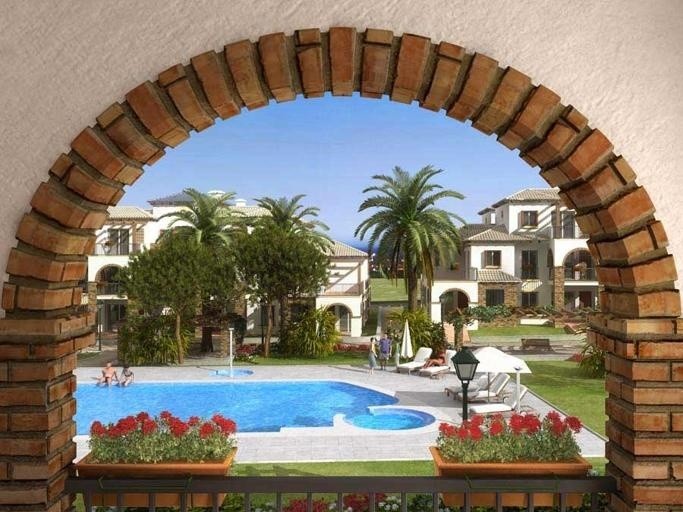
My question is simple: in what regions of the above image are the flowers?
[87,411,237,464]
[436,411,583,463]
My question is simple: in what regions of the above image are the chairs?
[397,346,433,375]
[419,350,457,379]
[445,373,528,415]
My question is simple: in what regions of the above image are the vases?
[429,446,593,507]
[76,447,238,508]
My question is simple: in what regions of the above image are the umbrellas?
[449,342,532,404]
[400,319,414,363]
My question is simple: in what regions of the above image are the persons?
[424,343,446,368]
[98,361,119,387]
[118,364,134,387]
[367,333,400,376]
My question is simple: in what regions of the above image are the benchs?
[521,339,551,352]
